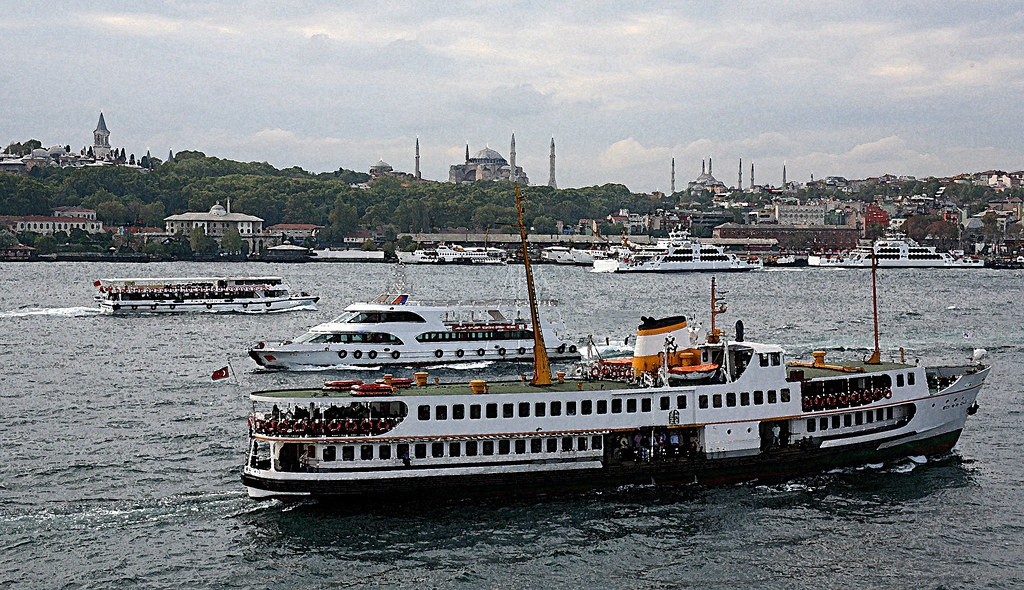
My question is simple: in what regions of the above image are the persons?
[402,448,411,470]
[606,431,704,459]
[771,424,780,446]
[272,403,398,419]
[299,450,308,471]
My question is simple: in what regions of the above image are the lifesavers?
[338,348,377,360]
[132,302,273,310]
[252,420,391,432]
[392,350,400,359]
[376,378,412,389]
[456,347,526,358]
[801,387,893,410]
[557,345,576,354]
[321,379,363,390]
[347,384,394,396]
[435,349,443,358]
[115,288,269,294]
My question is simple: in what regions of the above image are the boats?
[249,245,583,371]
[541,245,608,265]
[242,178,994,507]
[807,217,986,269]
[671,364,718,380]
[393,243,509,264]
[592,221,764,273]
[93,272,321,313]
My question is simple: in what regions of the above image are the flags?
[210,366,230,381]
[94,281,101,286]
